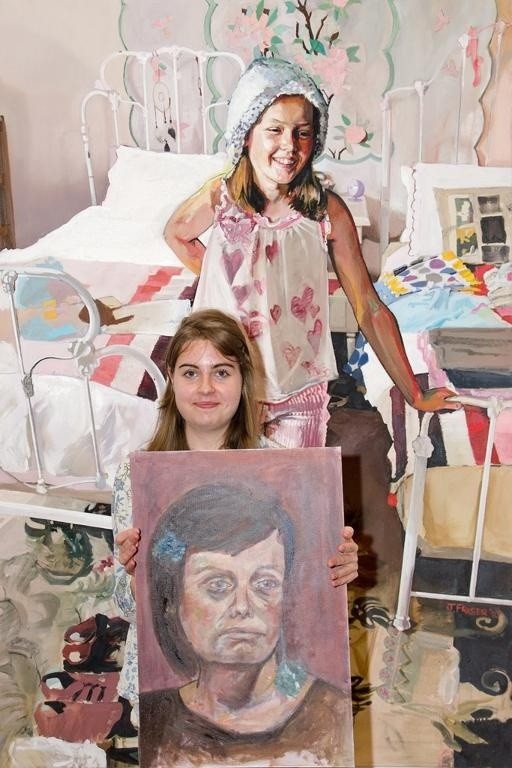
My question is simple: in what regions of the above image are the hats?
[222,54,331,168]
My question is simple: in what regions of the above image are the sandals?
[59,635,124,673]
[64,613,128,643]
[35,671,115,704]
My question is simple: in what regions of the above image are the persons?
[102,307,364,731]
[137,472,348,768]
[149,56,468,453]
